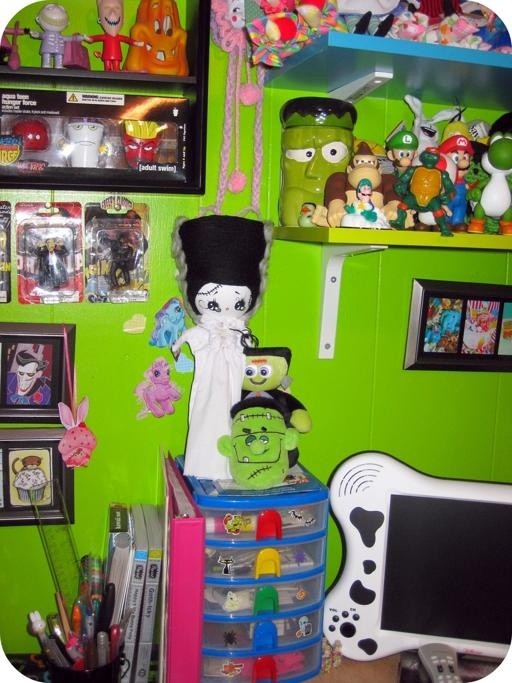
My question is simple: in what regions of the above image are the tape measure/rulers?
[26,475,89,626]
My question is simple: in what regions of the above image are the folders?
[160,447,205,683]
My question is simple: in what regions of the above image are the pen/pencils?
[28,580,121,672]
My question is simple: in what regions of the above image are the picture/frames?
[402,278,512,372]
[0,323,76,424]
[0,428,74,526]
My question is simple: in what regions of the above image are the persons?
[275,0,512,239]
[214,397,299,490]
[103,234,136,292]
[36,236,69,298]
[167,215,274,480]
[0,0,512,305]
[238,346,314,468]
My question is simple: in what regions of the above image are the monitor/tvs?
[324,450,512,662]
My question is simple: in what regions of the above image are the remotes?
[417,643,463,683]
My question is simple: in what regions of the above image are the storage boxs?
[176,452,331,683]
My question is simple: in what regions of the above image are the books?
[133,502,162,682]
[102,502,134,627]
[117,502,148,682]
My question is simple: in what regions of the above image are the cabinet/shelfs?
[262,31,512,252]
[1,0,212,196]
